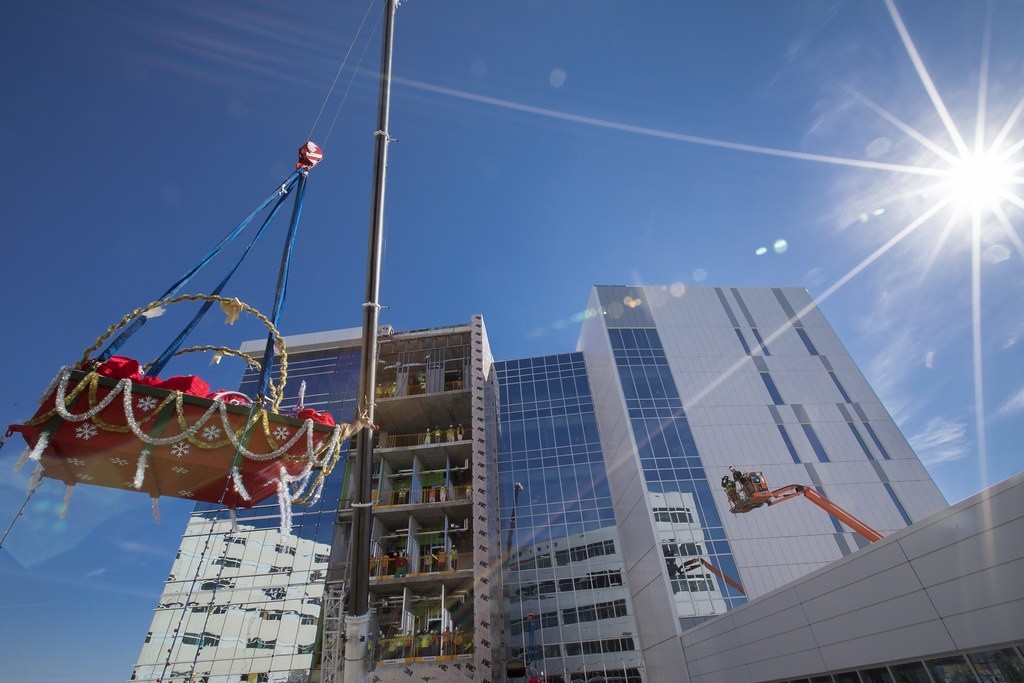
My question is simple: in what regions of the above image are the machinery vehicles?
[725,470,883,544]
[676,558,745,594]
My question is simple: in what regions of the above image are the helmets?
[724,476,729,479]
[368,625,450,635]
[729,466,735,471]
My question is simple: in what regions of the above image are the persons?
[370,545,458,576]
[423,424,464,444]
[419,379,425,394]
[721,465,748,507]
[398,480,472,504]
[375,381,396,398]
[367,625,465,664]
[455,370,462,382]
